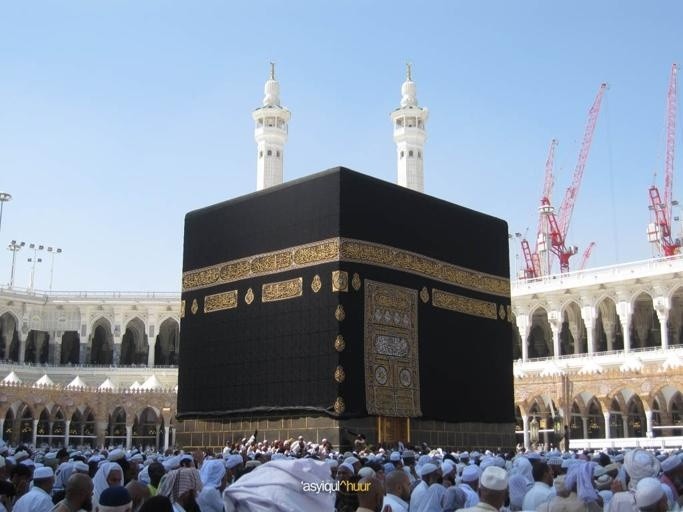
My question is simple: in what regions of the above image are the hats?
[33,467,54,479]
[339,463,355,474]
[66,472,95,486]
[13,449,103,466]
[441,463,454,477]
[334,447,491,462]
[421,463,438,476]
[225,454,244,468]
[480,466,509,491]
[661,455,682,472]
[99,486,133,507]
[515,452,625,489]
[634,478,665,507]
[108,448,125,461]
[461,467,478,483]
[180,454,194,462]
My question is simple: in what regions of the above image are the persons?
[0,429,682,512]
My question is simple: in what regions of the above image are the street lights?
[8,240,26,288]
[26,243,44,290]
[0,191,12,222]
[47,246,62,292]
